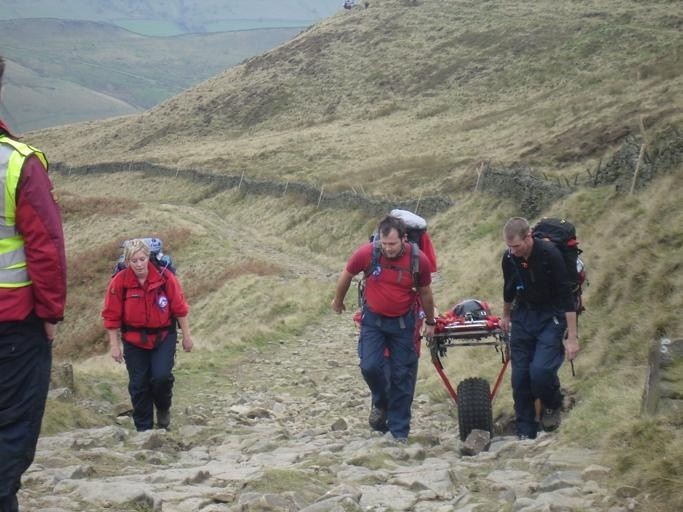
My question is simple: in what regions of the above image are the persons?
[100,238,195,432]
[497,216,580,439]
[330,214,439,443]
[0,55,69,512]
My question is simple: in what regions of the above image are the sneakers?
[157,405,171,428]
[368,403,388,428]
[540,404,562,431]
[394,434,407,447]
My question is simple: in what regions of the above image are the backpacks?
[508,218,588,320]
[109,238,177,350]
[357,209,431,315]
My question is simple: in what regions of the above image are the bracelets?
[424,319,436,325]
